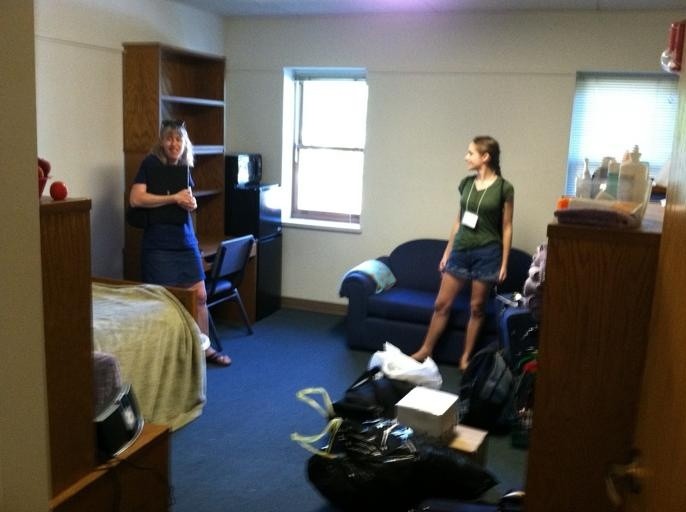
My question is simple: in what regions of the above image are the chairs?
[202,233,257,352]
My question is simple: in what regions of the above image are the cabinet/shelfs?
[520,199,665,512]
[122,40,224,286]
[40,193,98,497]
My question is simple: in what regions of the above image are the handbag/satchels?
[329,366,416,423]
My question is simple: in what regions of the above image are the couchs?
[339,237,531,367]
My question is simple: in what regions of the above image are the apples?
[38,168,44,179]
[49,181,69,201]
[38,157,51,176]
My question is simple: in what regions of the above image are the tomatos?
[50,181,67,200]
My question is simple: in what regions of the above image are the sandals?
[205,349,232,367]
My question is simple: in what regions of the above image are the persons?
[128,118,232,368]
[409,136,514,374]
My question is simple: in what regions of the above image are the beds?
[93,278,206,462]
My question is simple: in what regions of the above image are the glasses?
[161,119,186,129]
